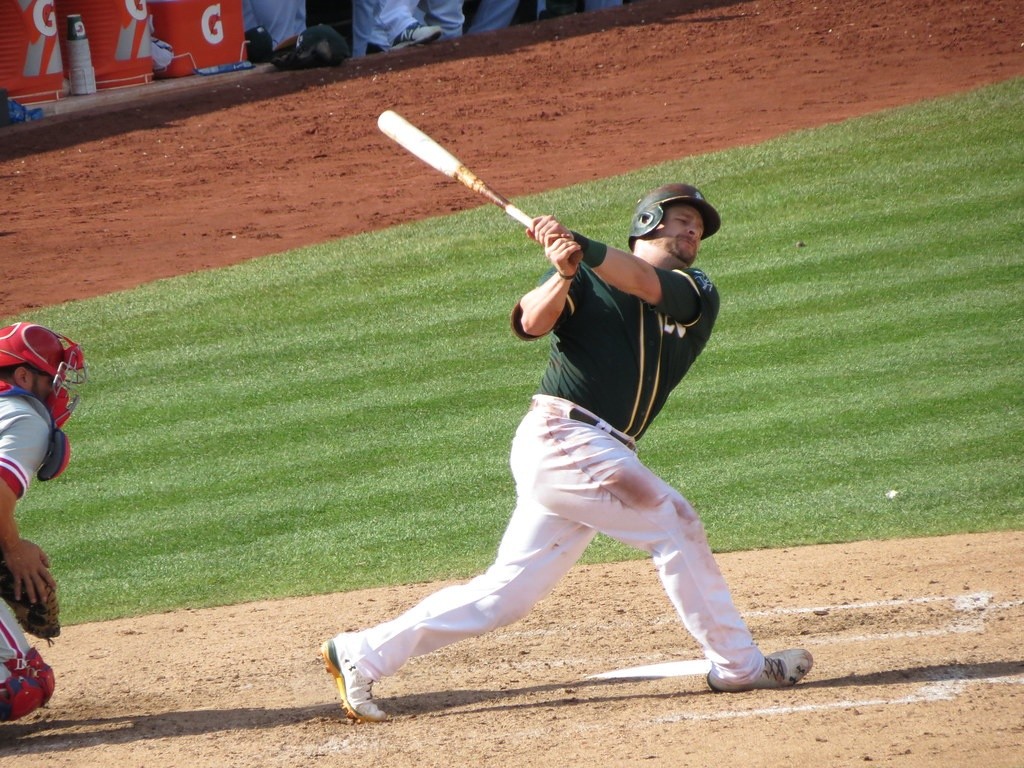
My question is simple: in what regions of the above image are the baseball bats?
[375,105,586,268]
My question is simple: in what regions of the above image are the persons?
[242,0,625,59]
[318,182,812,723]
[0,322,85,722]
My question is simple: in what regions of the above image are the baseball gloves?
[1,555,63,649]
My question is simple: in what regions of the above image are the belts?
[529,399,636,452]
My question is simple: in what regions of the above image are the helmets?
[629,183,721,252]
[290,24,352,69]
[0,322,66,381]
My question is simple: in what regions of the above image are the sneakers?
[391,22,441,51]
[316,635,387,725]
[707,649,814,693]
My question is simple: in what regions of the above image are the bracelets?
[569,230,607,268]
[557,266,578,280]
